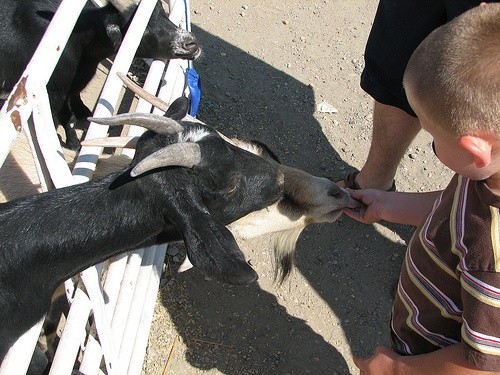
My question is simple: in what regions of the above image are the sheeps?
[0,0,205,153]
[0,69,354,375]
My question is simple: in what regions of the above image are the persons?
[334,0,500,209]
[343,0,499,375]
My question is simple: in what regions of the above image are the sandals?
[329,171,399,199]
